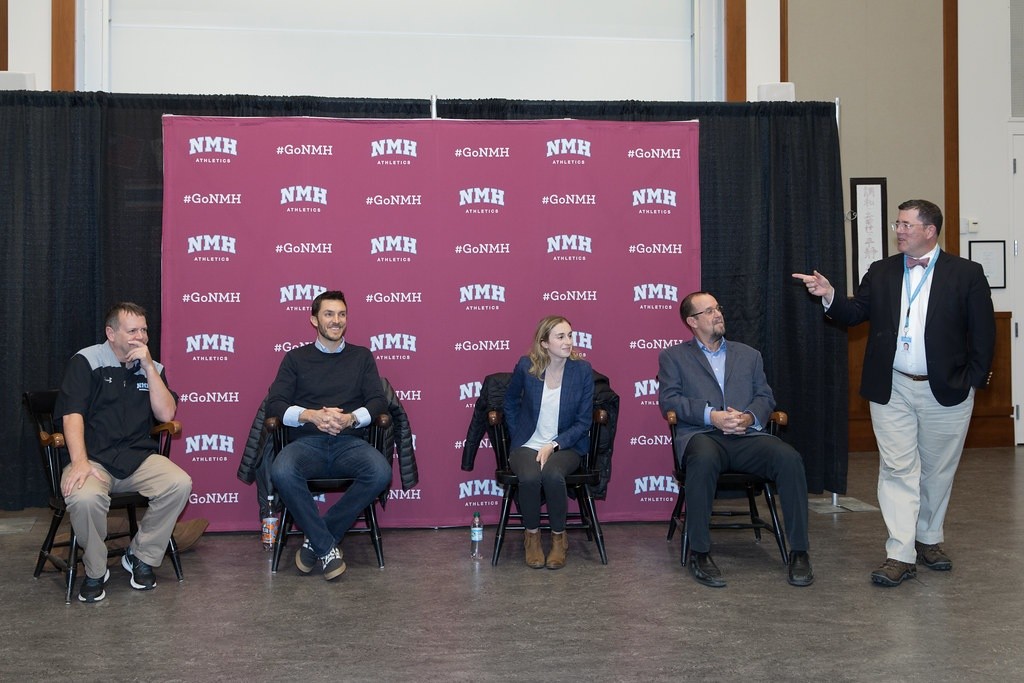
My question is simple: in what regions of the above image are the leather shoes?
[689,549,726,587]
[789,548,815,588]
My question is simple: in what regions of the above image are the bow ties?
[905,256,929,269]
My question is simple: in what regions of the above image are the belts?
[902,371,930,382]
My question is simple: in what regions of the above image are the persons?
[793,200,998,584]
[658,292,814,587]
[266,291,394,580]
[502,316,594,569]
[903,343,909,350]
[52,303,193,603]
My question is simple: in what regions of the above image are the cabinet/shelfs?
[847,311,1011,454]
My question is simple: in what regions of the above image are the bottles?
[261,495,279,551]
[470,511,483,560]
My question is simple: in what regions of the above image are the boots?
[547,529,569,569]
[524,527,545,568]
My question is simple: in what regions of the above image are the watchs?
[350,414,356,429]
[550,441,560,452]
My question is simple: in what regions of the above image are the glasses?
[691,304,724,317]
[890,220,934,234]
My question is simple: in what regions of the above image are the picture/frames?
[850,176,888,296]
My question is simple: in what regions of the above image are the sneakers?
[319,548,345,579]
[122,549,157,590]
[871,556,917,586]
[296,536,317,574]
[79,567,110,602]
[915,537,952,571]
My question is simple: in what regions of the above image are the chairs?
[265,376,389,573]
[656,374,789,568]
[486,369,609,565]
[23,390,183,604]
